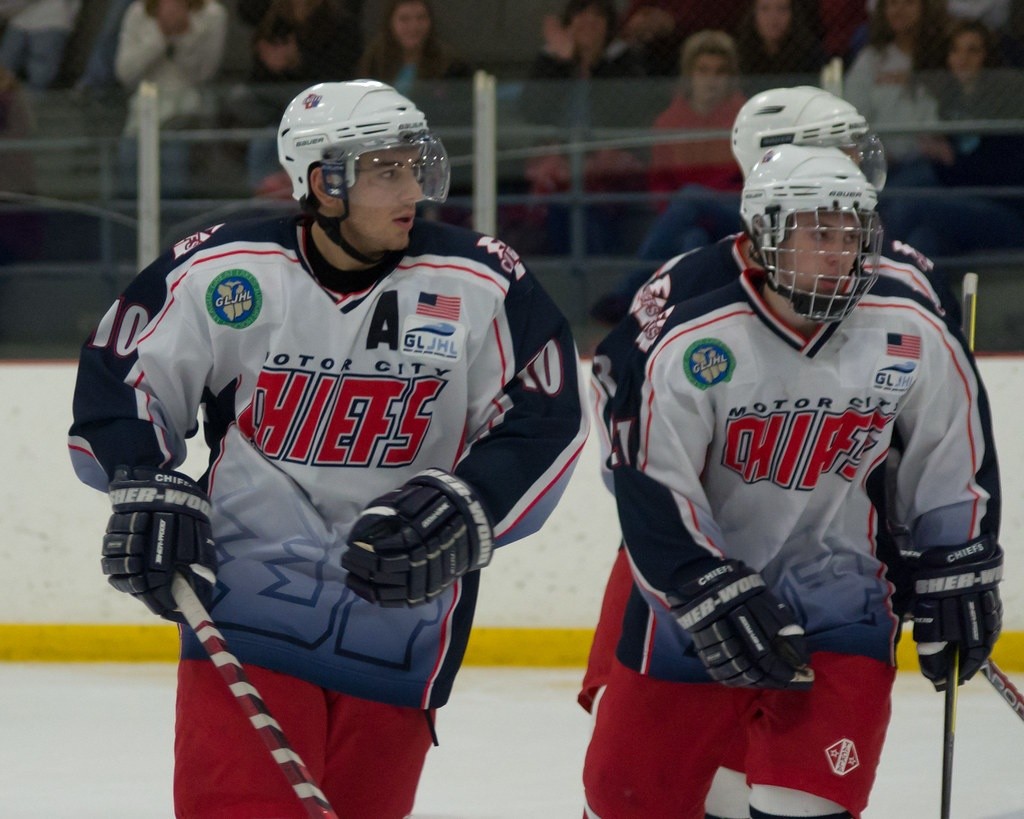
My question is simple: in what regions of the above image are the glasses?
[355,165,422,189]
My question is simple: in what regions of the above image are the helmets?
[740,144,883,323]
[279,78,449,205]
[731,85,888,192]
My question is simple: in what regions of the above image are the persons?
[574,80,966,710]
[3,4,1021,329]
[64,76,589,817]
[585,143,1003,819]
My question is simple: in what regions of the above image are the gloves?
[341,466,494,608]
[666,557,815,689]
[898,533,1004,692]
[101,464,215,625]
